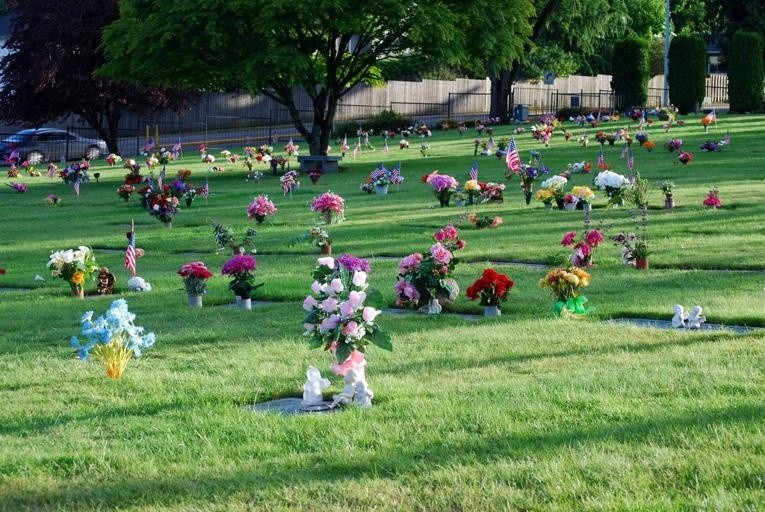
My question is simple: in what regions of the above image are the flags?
[0,107,730,278]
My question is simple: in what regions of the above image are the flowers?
[302,250,393,385]
[536,253,593,317]
[206,214,258,257]
[6,116,725,228]
[68,298,156,381]
[466,266,515,306]
[560,198,616,270]
[308,222,333,245]
[221,255,263,296]
[175,260,213,294]
[35,244,97,298]
[393,224,465,315]
[608,203,657,257]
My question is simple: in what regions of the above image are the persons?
[328,368,373,410]
[672,306,702,327]
[97,267,109,295]
[425,298,442,315]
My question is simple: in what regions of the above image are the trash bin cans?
[515,104,529,121]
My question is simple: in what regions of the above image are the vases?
[235,297,253,311]
[484,306,502,318]
[321,245,332,255]
[634,257,650,270]
[186,295,204,308]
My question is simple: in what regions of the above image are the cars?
[0,128,107,166]
